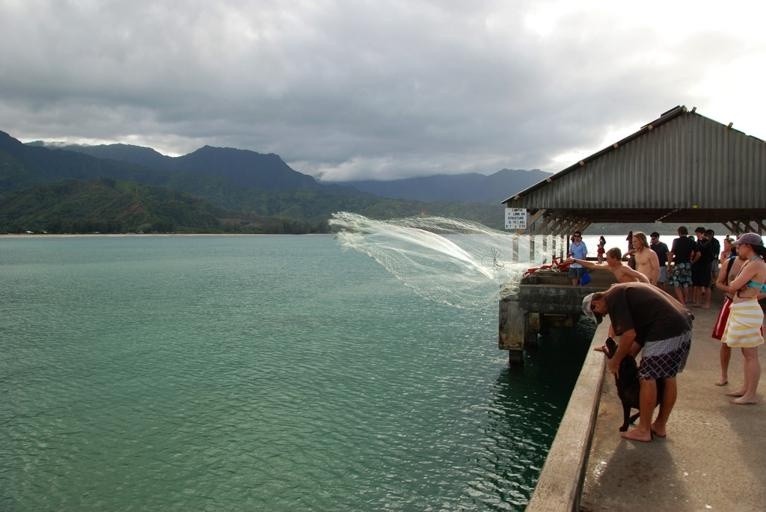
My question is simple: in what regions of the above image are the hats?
[731,233,762,247]
[582,293,603,325]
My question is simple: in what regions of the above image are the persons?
[692,227,720,309]
[631,232,660,286]
[668,226,701,307]
[582,282,695,441]
[597,236,606,264]
[568,231,588,286]
[712,233,766,404]
[566,248,651,285]
[623,249,637,271]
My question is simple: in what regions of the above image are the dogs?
[602,337,665,432]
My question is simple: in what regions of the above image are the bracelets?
[573,259,576,263]
